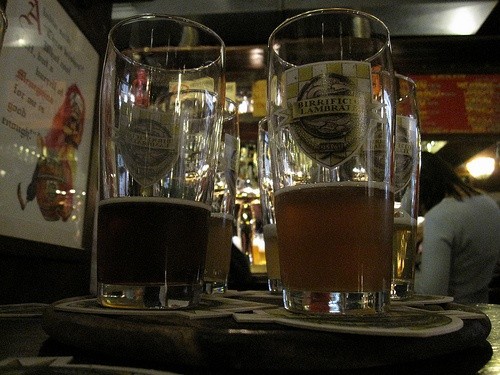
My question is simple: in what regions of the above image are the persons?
[228,243,254,290]
[413,151,500,305]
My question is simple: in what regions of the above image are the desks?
[0,296,500,375]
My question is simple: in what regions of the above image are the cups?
[365,71,421,300]
[257,116,283,297]
[152,90,241,307]
[245,205,267,277]
[95,15,225,310]
[266,8,395,315]
